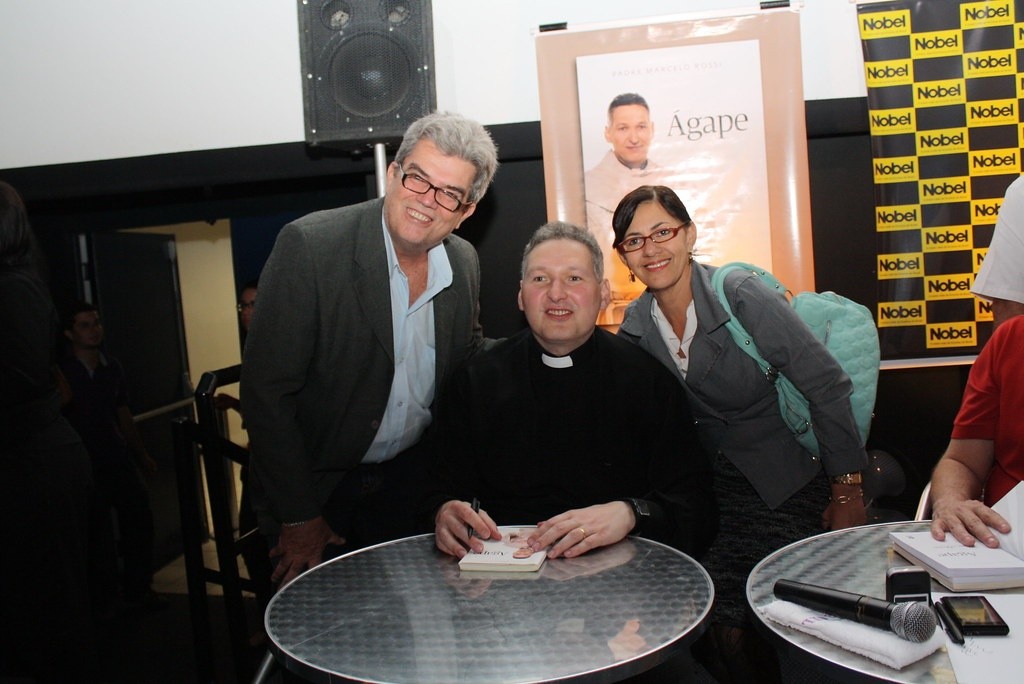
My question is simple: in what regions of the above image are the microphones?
[773,578,937,644]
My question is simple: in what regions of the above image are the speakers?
[296,0,437,156]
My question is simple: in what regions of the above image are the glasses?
[395,158,475,215]
[613,218,690,255]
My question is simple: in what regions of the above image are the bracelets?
[828,488,864,504]
[830,473,862,484]
[282,521,305,529]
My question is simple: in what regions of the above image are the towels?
[756,599,947,670]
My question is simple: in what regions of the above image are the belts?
[349,461,401,477]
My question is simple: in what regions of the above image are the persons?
[502,528,543,559]
[611,185,869,684]
[402,220,720,558]
[928,174,1024,547]
[584,93,663,285]
[236,111,507,605]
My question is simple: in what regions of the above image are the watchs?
[622,497,650,532]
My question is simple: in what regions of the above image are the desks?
[746,519,1024,684]
[264,525,715,684]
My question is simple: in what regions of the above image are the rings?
[578,528,586,539]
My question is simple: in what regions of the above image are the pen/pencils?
[468,498,479,538]
[936,602,964,643]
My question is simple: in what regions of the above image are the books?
[890,480,1024,592]
[459,525,550,572]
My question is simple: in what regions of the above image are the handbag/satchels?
[711,262,880,460]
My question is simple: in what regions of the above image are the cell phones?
[942,596,1010,636]
